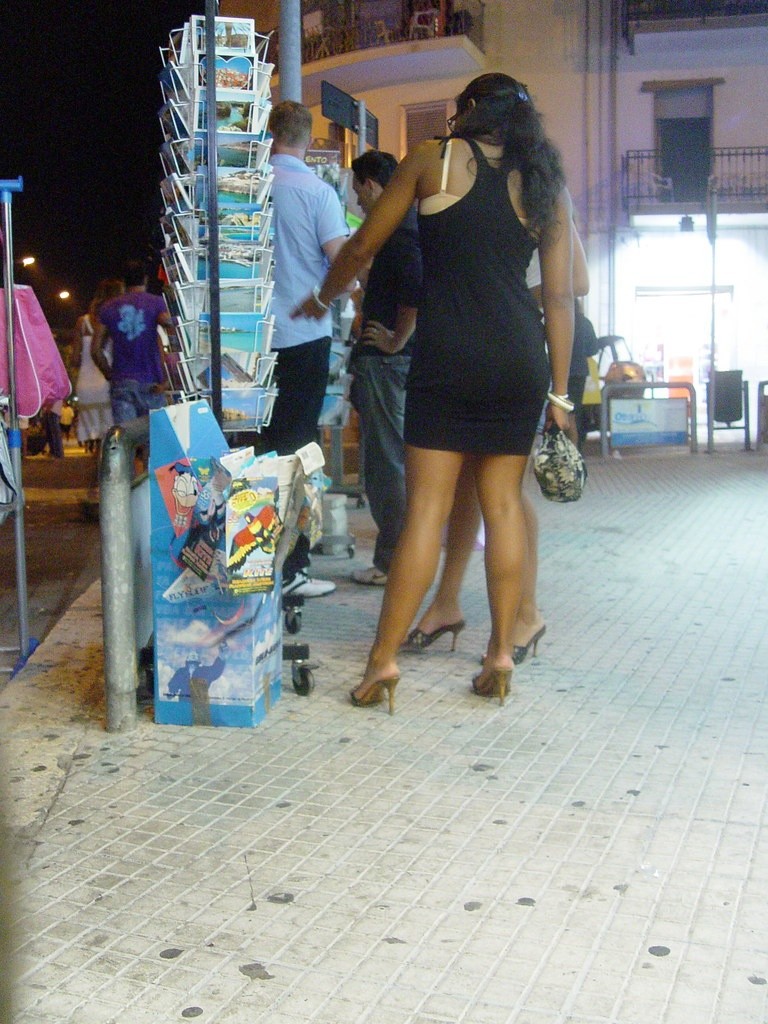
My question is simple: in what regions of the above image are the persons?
[403,221,589,664]
[91,263,177,479]
[237,101,355,597]
[291,73,576,717]
[351,149,419,584]
[42,399,65,473]
[568,298,599,458]
[71,282,126,496]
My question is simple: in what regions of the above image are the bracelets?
[311,286,328,311]
[546,392,574,413]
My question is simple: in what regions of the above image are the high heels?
[401,618,466,652]
[481,623,546,666]
[347,671,400,716]
[471,665,514,706]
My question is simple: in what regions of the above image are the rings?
[374,329,376,333]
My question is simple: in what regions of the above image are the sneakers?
[283,571,335,598]
[352,566,389,587]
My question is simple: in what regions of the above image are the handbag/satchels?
[0,283,72,418]
[533,418,586,503]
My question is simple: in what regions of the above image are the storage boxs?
[146,401,331,728]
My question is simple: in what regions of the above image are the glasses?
[448,107,469,135]
